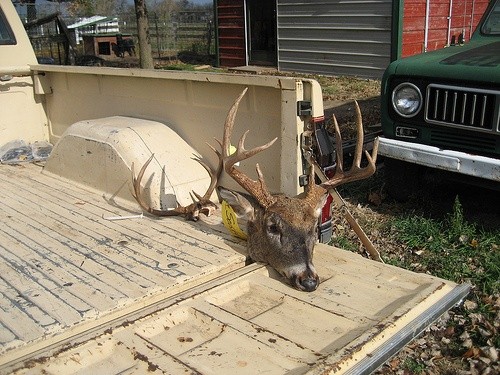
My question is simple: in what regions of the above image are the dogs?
[115,34,137,58]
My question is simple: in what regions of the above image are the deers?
[222,88,380,291]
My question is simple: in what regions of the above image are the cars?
[377,1,500,182]
